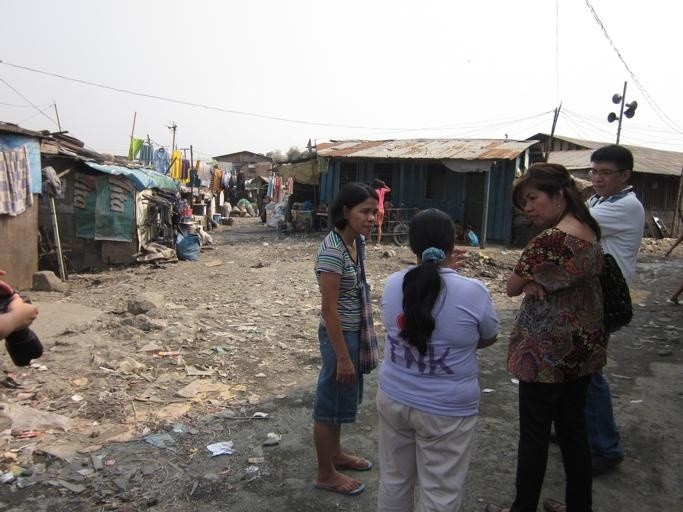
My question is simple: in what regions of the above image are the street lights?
[606,80,638,145]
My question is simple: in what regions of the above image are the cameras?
[0,292,43,367]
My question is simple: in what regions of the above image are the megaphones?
[624,109,635,118]
[612,94,622,103]
[607,112,619,122]
[626,101,638,109]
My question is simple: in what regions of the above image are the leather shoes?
[486,499,569,511]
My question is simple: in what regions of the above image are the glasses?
[589,169,618,177]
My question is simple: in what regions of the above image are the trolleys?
[369,207,419,247]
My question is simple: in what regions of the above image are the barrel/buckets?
[184,216,192,222]
[213,213,222,225]
[183,206,193,216]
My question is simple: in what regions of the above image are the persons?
[484,162,609,512]
[365,177,391,248]
[310,181,379,498]
[0,270,39,340]
[373,206,499,512]
[550,144,647,478]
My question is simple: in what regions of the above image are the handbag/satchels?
[600,252,632,333]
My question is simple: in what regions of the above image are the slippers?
[334,455,372,471]
[313,471,365,495]
